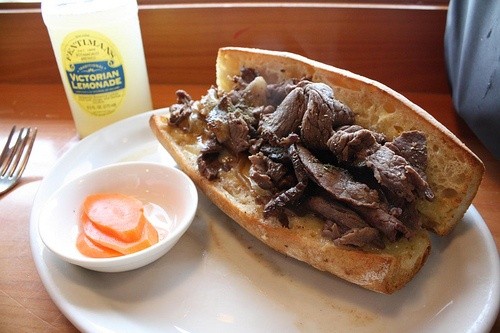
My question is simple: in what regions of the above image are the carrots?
[77,233,123,258]
[83,192,146,243]
[83,212,158,255]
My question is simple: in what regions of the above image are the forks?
[0,125,37,195]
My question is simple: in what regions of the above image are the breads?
[149,47,486,294]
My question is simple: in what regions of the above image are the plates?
[30,107,500,332]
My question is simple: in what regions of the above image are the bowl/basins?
[36,162,198,270]
